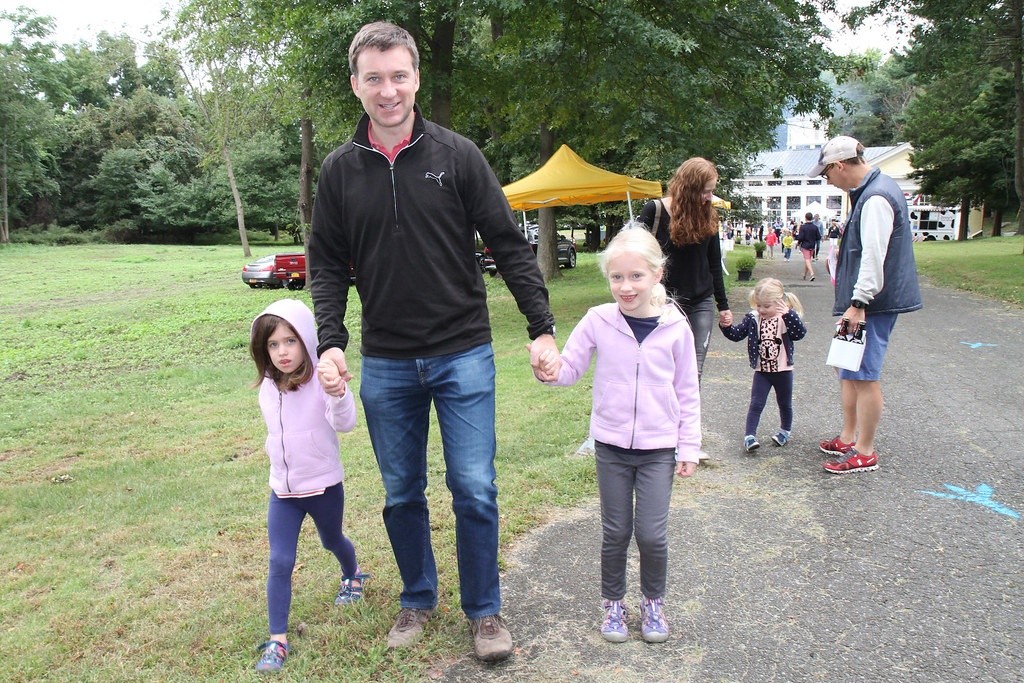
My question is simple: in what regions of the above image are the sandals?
[254,638,289,673]
[334,565,370,605]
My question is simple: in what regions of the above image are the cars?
[241,251,306,291]
[483,222,577,276]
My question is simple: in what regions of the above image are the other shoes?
[816,254,818,260]
[784,258,790,261]
[809,275,815,281]
[674,447,710,461]
[803,275,805,279]
[812,257,815,261]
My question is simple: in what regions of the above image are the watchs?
[851,300,868,310]
[542,325,557,338]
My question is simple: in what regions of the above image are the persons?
[637,156,732,461]
[807,135,923,475]
[795,212,821,282]
[813,214,824,261]
[722,217,797,263]
[247,299,369,675]
[718,277,807,449]
[827,219,844,253]
[309,20,563,662]
[525,215,701,643]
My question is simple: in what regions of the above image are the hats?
[807,135,859,178]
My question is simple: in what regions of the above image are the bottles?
[834,318,849,341]
[849,320,867,345]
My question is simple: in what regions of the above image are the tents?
[502,142,662,240]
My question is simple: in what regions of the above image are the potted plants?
[736,237,743,244]
[754,240,766,258]
[736,254,756,281]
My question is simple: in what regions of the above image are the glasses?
[822,163,834,179]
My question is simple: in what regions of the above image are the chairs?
[720,239,729,275]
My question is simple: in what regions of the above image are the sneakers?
[772,432,789,447]
[743,437,761,452]
[822,448,880,474]
[467,611,513,662]
[600,597,630,642]
[387,605,436,648]
[819,436,855,456]
[639,595,669,642]
